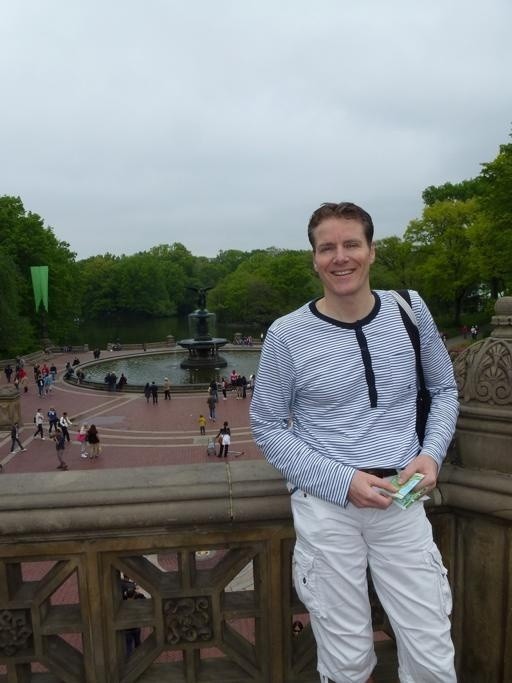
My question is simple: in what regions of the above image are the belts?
[359,468,397,478]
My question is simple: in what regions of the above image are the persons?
[462,324,467,338]
[122,578,146,661]
[197,287,208,310]
[245,199,464,682]
[0,327,267,471]
[474,324,480,340]
[470,324,475,339]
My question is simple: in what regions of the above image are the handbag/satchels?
[393,289,461,454]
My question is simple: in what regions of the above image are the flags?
[28,266,50,314]
